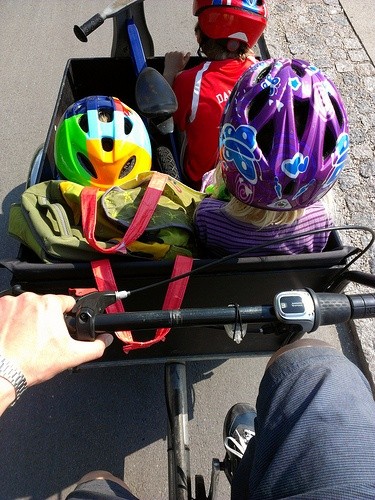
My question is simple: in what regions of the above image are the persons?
[0,291,375,500]
[189,58,349,258]
[157,0,269,186]
[47,96,152,191]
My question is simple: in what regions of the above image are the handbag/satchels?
[79,169,215,354]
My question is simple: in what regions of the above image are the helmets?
[216,56,352,213]
[52,94,154,192]
[191,0,270,50]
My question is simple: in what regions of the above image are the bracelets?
[0,354,29,407]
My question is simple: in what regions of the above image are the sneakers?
[220,401,261,478]
